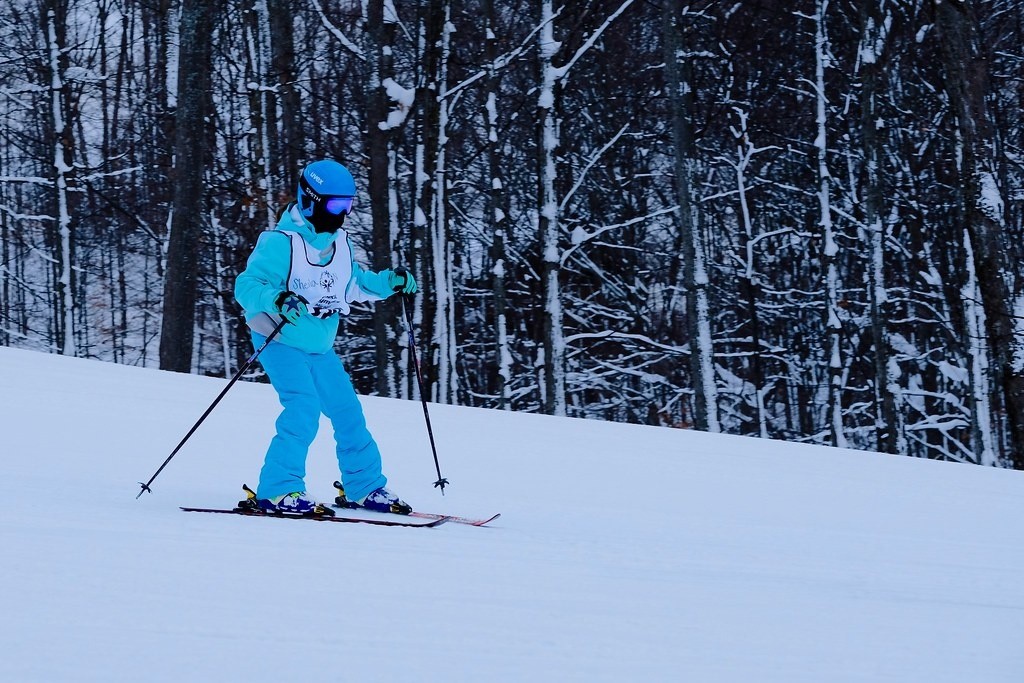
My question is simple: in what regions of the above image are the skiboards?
[178,501,502,529]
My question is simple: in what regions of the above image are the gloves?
[274,291,308,326]
[388,271,417,303]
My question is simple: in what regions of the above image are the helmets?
[297,160,356,235]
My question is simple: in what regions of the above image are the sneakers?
[335,481,412,515]
[257,492,335,517]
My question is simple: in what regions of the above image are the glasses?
[326,197,354,215]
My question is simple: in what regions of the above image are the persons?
[235,160,417,513]
